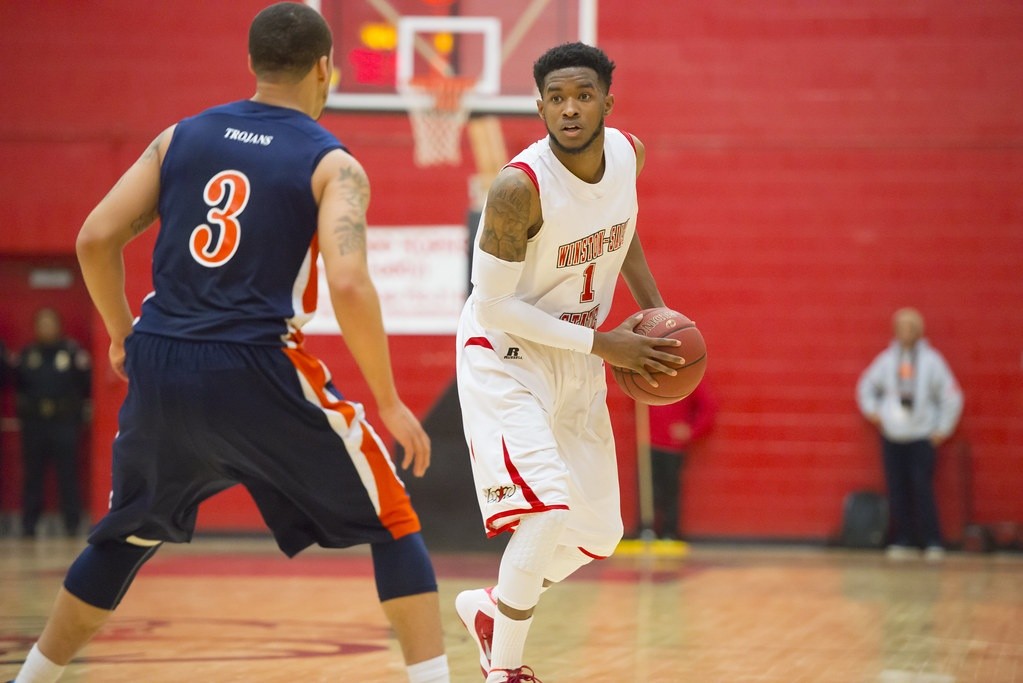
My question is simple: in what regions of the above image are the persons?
[455,40,687,683]
[11,304,94,540]
[856,309,964,562]
[13,0,449,682]
[635,377,715,542]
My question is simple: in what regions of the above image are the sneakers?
[485,665,541,683]
[455,585,501,677]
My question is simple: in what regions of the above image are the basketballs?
[610,308,707,407]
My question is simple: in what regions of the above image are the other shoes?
[886,545,925,560]
[924,546,946,565]
